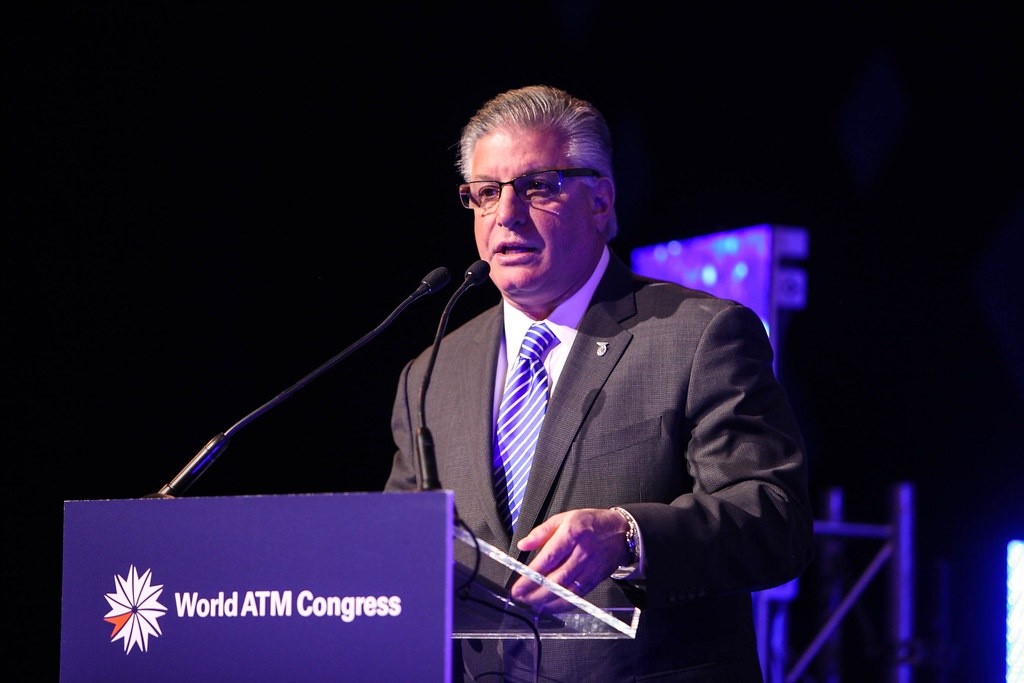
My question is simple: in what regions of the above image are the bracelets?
[610,505,641,567]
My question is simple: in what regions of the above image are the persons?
[378,83,817,682]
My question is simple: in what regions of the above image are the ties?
[493,323,560,540]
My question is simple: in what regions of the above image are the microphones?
[144,266,450,499]
[414,261,491,492]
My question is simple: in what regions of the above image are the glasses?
[458,168,601,210]
